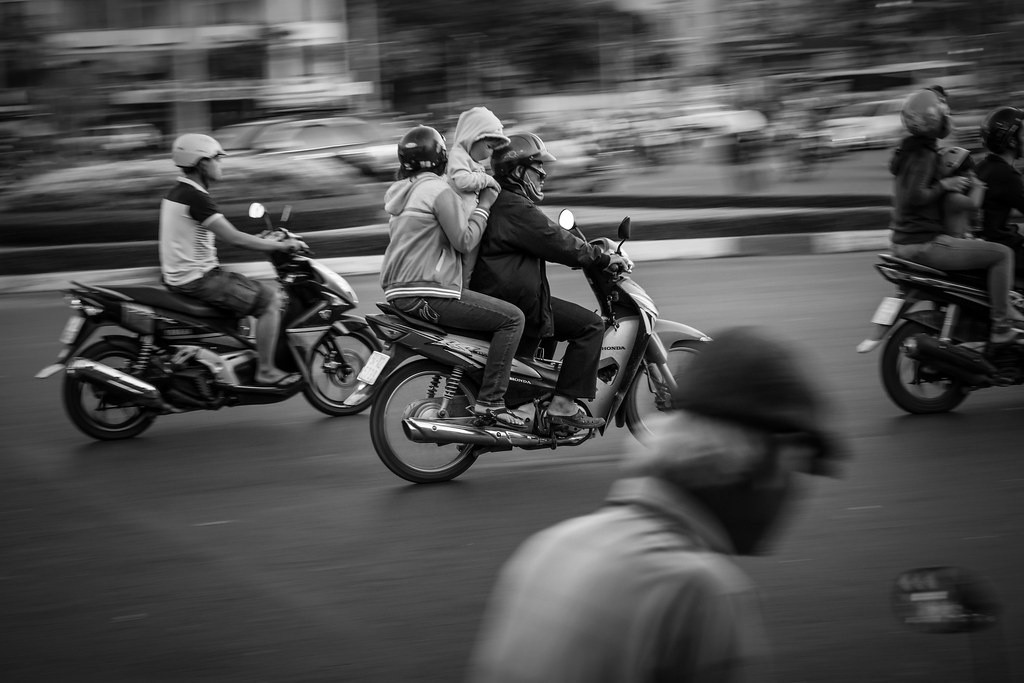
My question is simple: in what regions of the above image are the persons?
[890,84,1024,343]
[445,105,511,289]
[968,107,1024,295]
[159,133,310,388]
[382,124,528,432]
[465,324,855,683]
[469,134,634,428]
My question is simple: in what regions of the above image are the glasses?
[523,164,547,182]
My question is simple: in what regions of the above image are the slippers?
[547,409,606,429]
[474,406,528,429]
[983,329,1023,354]
[252,371,305,390]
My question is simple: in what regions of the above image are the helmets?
[671,326,856,460]
[979,106,1023,156]
[899,85,952,139]
[490,132,556,168]
[170,132,228,168]
[397,124,448,170]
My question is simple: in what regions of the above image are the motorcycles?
[35,203,384,441]
[342,209,713,484]
[855,253,1024,414]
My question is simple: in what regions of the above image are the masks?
[521,171,545,205]
